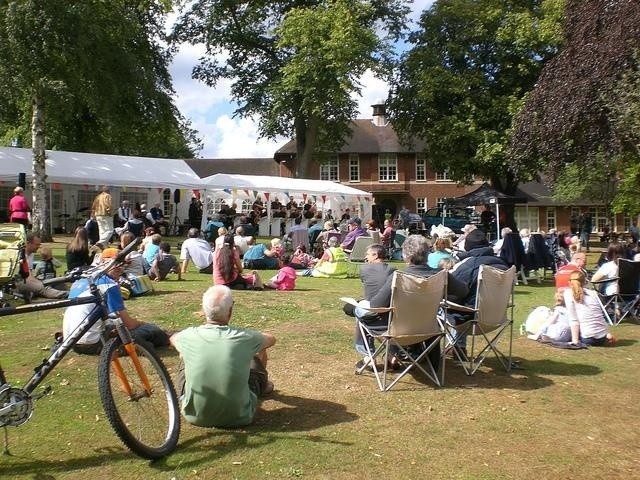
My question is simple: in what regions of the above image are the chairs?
[327,233,341,247]
[355,269,448,392]
[525,234,553,283]
[500,232,527,285]
[235,224,253,237]
[310,230,322,252]
[592,259,640,325]
[383,230,396,259]
[205,224,225,241]
[343,237,375,276]
[286,229,308,250]
[128,221,141,237]
[441,266,516,375]
[371,232,381,245]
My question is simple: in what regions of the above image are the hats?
[348,217,362,225]
[14,186,24,192]
[460,224,470,232]
[572,252,585,261]
[464,229,490,251]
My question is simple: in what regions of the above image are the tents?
[444,183,537,240]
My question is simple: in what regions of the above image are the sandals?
[607,336,615,347]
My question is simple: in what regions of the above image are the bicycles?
[0,237,180,457]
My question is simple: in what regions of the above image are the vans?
[421,208,481,233]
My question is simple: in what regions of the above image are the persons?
[10,232,70,298]
[60,186,179,356]
[170,284,276,429]
[572,212,591,253]
[479,204,507,229]
[527,253,613,345]
[308,208,377,279]
[344,225,508,370]
[10,187,32,230]
[181,217,314,290]
[375,209,426,250]
[188,196,316,229]
[592,224,640,315]
[492,227,578,256]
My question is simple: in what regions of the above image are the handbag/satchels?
[344,299,386,326]
[130,274,152,296]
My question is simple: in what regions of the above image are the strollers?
[0,223,33,308]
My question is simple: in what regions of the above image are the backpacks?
[44,261,56,279]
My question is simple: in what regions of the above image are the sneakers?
[355,360,384,377]
